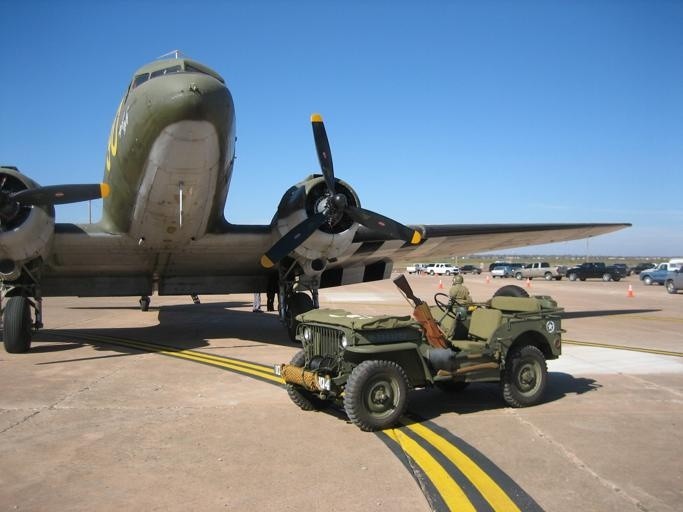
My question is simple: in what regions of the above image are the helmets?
[453,275,463,285]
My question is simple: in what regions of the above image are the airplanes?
[0,52,629,357]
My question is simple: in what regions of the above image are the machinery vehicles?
[283,274,567,430]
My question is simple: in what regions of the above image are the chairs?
[429,306,457,340]
[452,308,503,354]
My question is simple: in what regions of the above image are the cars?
[405,259,683,296]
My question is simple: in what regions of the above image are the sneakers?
[253,309,264,312]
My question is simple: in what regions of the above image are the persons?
[446,275,472,303]
[265,293,275,311]
[251,293,263,312]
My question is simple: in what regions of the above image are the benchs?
[490,296,541,311]
